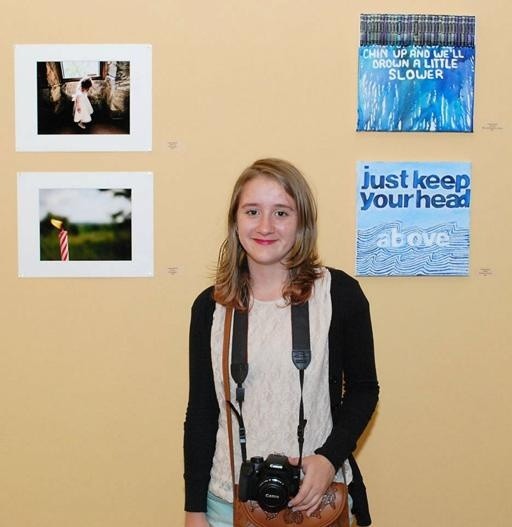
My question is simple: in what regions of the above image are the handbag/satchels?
[233,483,350,527]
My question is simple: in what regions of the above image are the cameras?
[238,455,301,514]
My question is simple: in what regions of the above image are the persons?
[73,78,94,129]
[185,159,379,527]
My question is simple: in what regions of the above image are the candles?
[49,216,72,260]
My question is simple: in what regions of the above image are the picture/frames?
[15,45,152,154]
[19,172,155,276]
